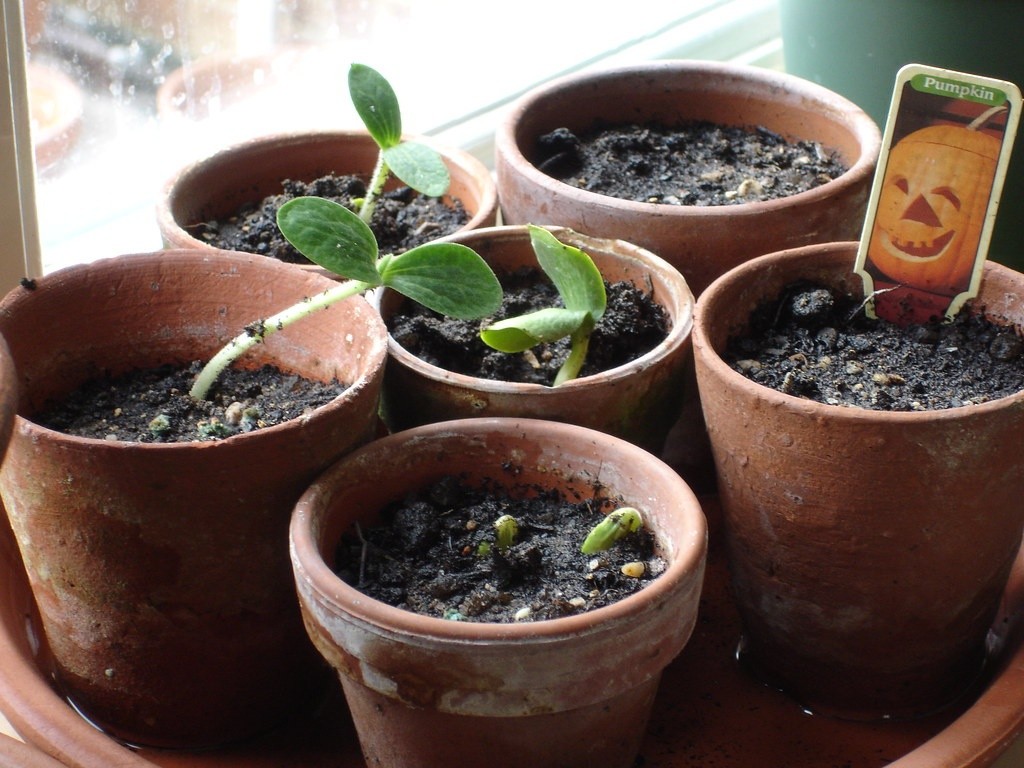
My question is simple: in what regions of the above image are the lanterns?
[868,102,1006,293]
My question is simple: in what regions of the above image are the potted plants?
[4,54,1021,768]
